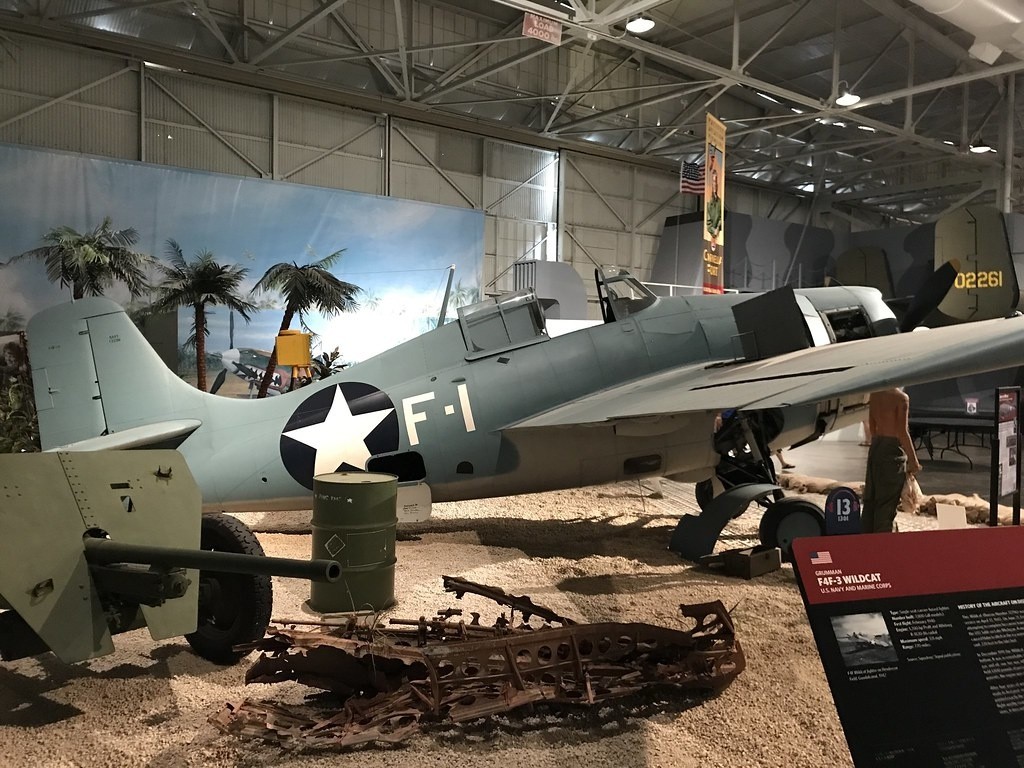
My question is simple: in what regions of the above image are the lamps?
[626,15,655,33]
[969,131,990,153]
[835,80,861,107]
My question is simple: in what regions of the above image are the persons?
[714,413,722,434]
[707,175,722,237]
[776,453,795,469]
[3,341,27,383]
[861,386,920,533]
[859,420,871,446]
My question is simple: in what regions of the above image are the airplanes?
[0,262,1024,567]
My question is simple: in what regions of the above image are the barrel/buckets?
[311,472,399,612]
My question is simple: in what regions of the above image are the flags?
[680,162,705,194]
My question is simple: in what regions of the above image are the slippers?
[782,464,795,469]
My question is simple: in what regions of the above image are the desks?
[906,406,998,470]
